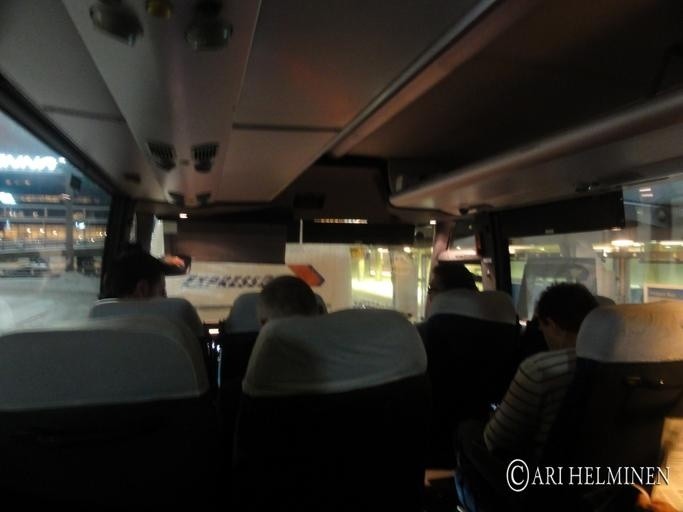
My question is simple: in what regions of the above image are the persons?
[96,251,166,300]
[253,275,322,328]
[598,481,679,512]
[453,282,600,511]
[155,252,186,270]
[424,259,484,320]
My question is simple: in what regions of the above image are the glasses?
[428,288,439,296]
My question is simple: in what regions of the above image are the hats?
[101,246,186,299]
